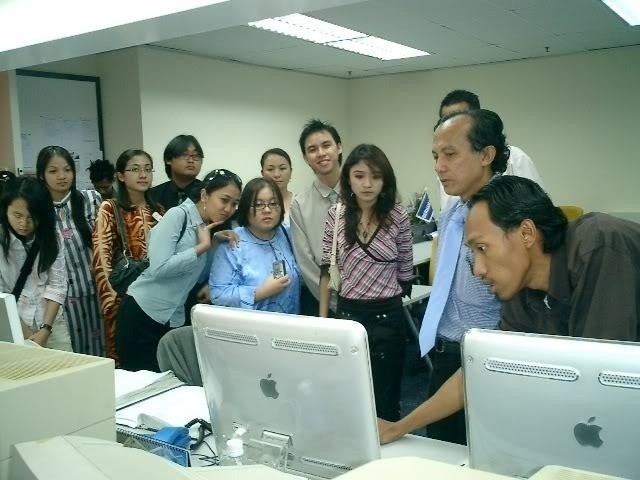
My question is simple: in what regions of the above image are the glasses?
[251,202,280,209]
[205,168,242,187]
[123,166,155,173]
[175,153,201,161]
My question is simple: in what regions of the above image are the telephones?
[146,418,213,451]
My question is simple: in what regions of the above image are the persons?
[436,89,544,213]
[418,108,510,446]
[148,135,203,212]
[320,143,422,422]
[377,175,640,445]
[34,145,104,359]
[93,149,165,368]
[0,177,73,353]
[209,177,301,316]
[88,159,117,200]
[261,148,295,226]
[114,169,242,371]
[289,119,344,314]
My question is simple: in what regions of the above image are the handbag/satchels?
[109,198,133,269]
[327,202,341,292]
[108,206,187,294]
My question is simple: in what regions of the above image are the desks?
[401,284,433,371]
[113,367,468,480]
[412,239,434,286]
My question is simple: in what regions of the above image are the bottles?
[219,439,247,466]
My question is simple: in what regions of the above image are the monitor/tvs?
[190,303,381,480]
[0,292,25,346]
[461,328,640,479]
[11,433,306,480]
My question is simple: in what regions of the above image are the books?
[114,368,186,411]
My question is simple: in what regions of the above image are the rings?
[225,236,227,240]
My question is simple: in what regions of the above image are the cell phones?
[273,260,286,279]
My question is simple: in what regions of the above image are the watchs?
[40,323,53,335]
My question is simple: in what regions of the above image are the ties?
[419,202,465,357]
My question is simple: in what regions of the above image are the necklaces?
[356,208,375,243]
